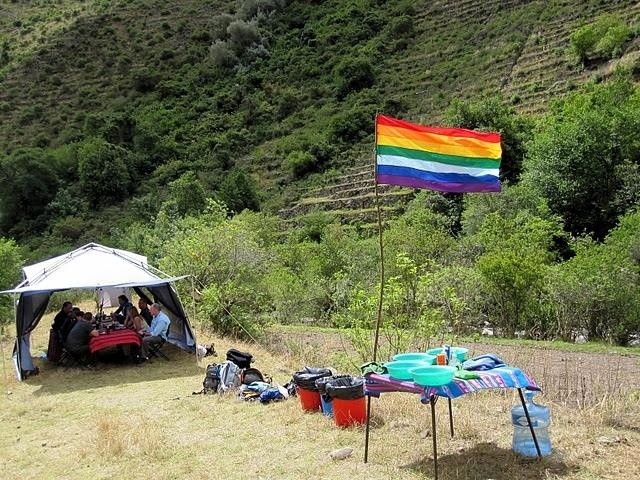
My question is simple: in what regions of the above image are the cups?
[437,353,446,365]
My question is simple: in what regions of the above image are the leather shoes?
[83,364,96,368]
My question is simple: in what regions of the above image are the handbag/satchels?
[262,372,273,384]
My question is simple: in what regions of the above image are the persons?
[52,301,100,368]
[114,294,133,317]
[138,298,152,328]
[124,306,148,331]
[140,303,171,359]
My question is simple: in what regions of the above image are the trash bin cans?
[293,367,366,429]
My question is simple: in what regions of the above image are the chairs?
[52,307,171,371]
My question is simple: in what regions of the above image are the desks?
[359,364,544,480]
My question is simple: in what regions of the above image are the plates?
[91,315,126,334]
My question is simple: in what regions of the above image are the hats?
[76,311,85,316]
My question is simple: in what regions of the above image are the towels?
[360,360,386,377]
[454,364,480,379]
[462,353,505,370]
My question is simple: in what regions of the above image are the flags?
[377,114,503,194]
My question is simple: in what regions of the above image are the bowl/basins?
[391,352,435,364]
[408,365,458,386]
[384,361,422,381]
[427,347,468,364]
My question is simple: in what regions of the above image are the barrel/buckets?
[511,390,552,458]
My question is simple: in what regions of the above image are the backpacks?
[192,363,223,395]
[240,390,260,402]
[216,360,242,395]
[226,349,255,369]
[241,368,264,385]
